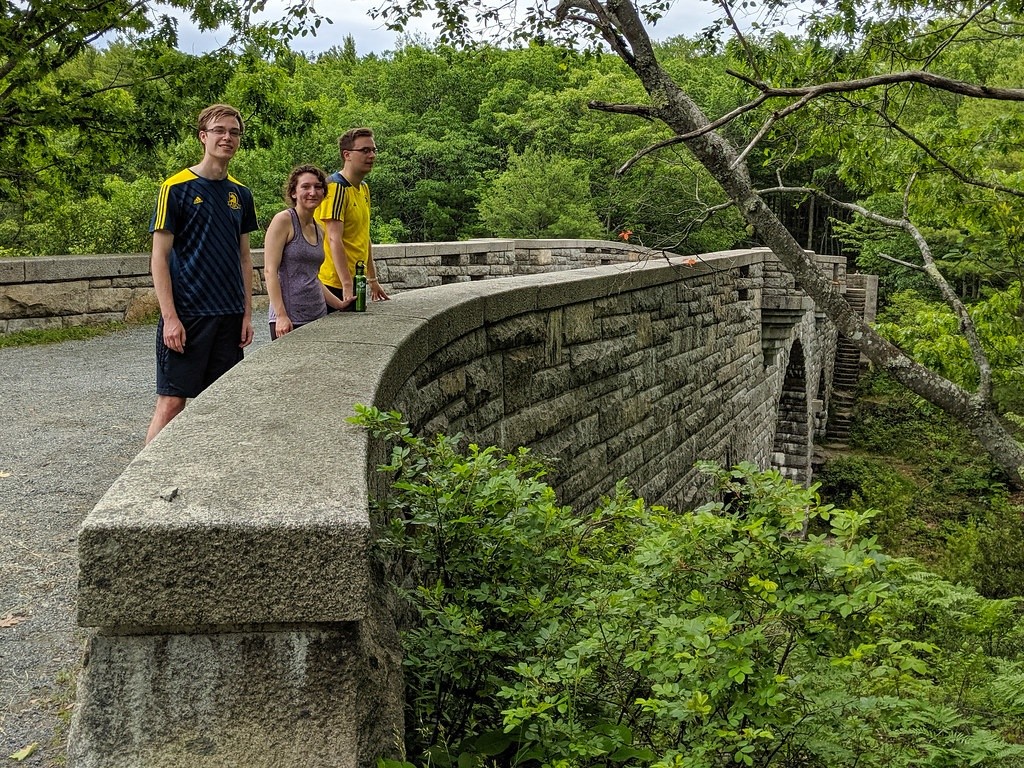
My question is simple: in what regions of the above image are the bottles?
[352,260,366,312]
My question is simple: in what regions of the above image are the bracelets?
[367,277,377,281]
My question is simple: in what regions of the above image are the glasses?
[204,126,242,137]
[348,147,378,154]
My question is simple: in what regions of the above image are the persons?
[146,104,260,446]
[313,128,391,314]
[264,165,367,341]
